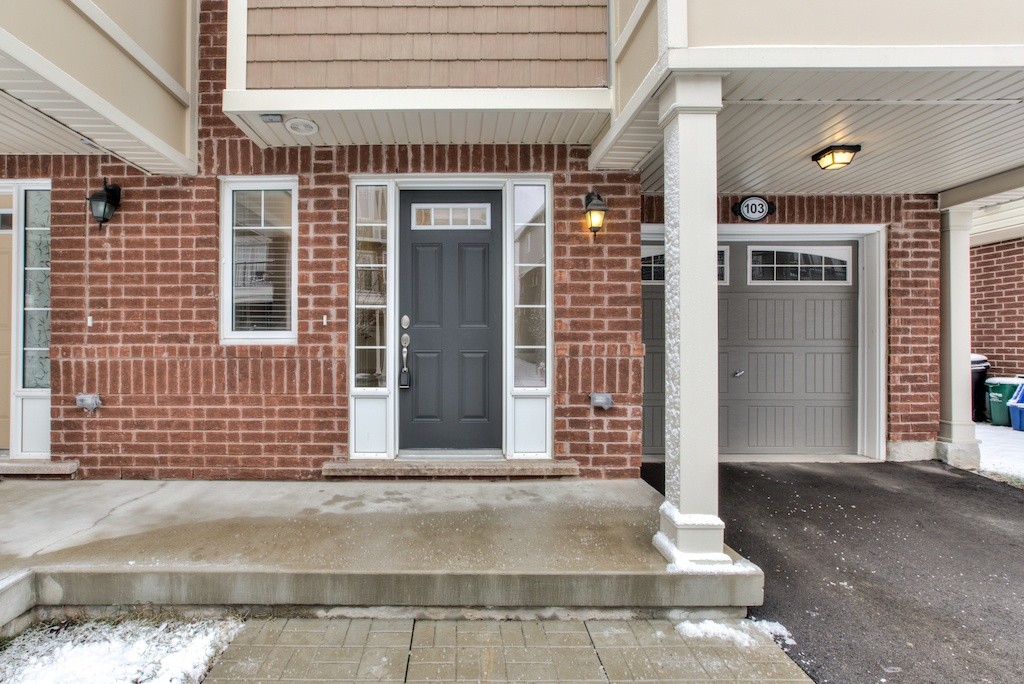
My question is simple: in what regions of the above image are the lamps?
[582,191,609,242]
[811,144,861,171]
[85,176,121,232]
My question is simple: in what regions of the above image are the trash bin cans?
[1007,383,1024,431]
[971,353,991,422]
[985,377,1023,426]
